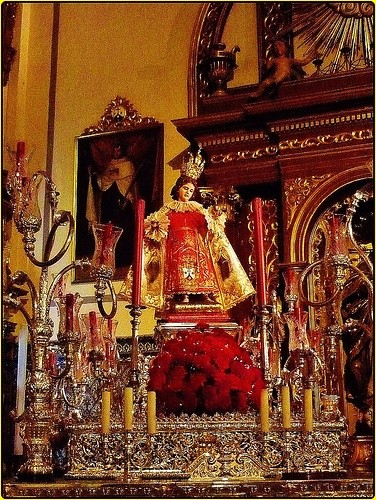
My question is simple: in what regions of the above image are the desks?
[3,476,375,497]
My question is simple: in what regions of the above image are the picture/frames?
[256,1,375,84]
[71,123,164,285]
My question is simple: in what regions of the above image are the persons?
[247,38,324,99]
[145,175,226,303]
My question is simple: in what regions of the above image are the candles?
[250,196,267,307]
[260,388,269,432]
[147,391,157,433]
[88,311,99,347]
[65,293,74,331]
[131,200,146,304]
[294,308,303,325]
[329,215,341,254]
[124,387,134,431]
[16,141,27,166]
[100,390,111,433]
[280,386,290,429]
[305,388,313,432]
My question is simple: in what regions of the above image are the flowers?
[147,323,268,412]
